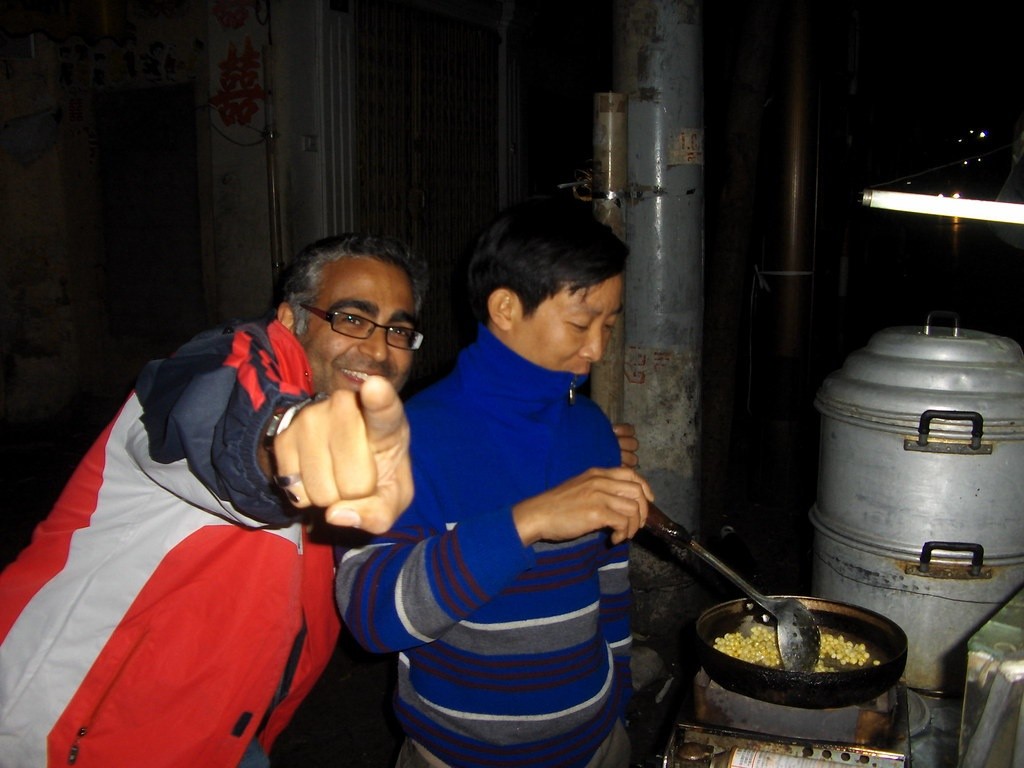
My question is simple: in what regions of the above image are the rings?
[275,473,300,488]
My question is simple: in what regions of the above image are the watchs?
[263,389,330,502]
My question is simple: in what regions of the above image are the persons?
[333,199,655,768]
[0,231,638,768]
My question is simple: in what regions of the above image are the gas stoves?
[676,666,911,768]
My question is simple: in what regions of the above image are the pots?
[695,594,909,710]
[807,311,1024,692]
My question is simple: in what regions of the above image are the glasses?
[300,303,424,352]
